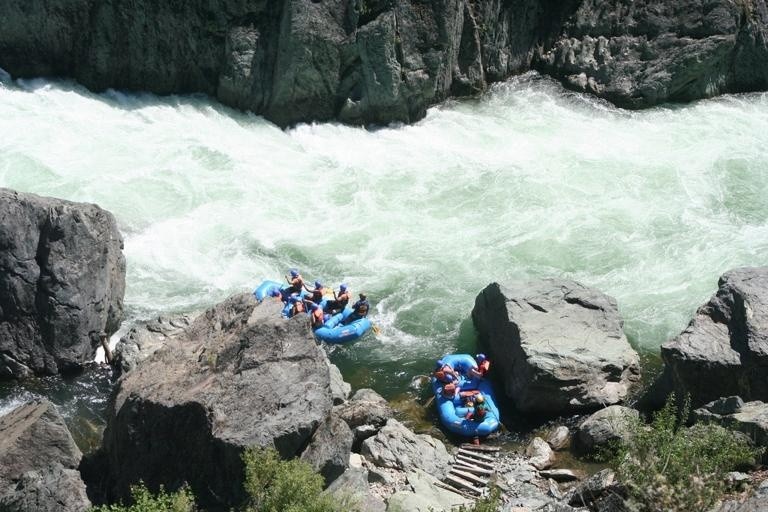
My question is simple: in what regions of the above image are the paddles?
[303,282,334,296]
[366,316,379,333]
[481,390,510,435]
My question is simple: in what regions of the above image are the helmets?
[315,281,321,288]
[476,395,484,404]
[272,271,312,302]
[341,285,346,290]
[311,303,318,309]
[359,293,366,299]
[476,353,486,363]
[436,360,444,367]
[444,374,454,382]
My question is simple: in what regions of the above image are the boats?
[432,353,503,436]
[253,280,373,343]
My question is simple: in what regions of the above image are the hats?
[465,413,473,420]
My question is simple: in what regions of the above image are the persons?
[309,303,324,329]
[429,359,460,385]
[300,279,324,304]
[351,293,369,321]
[439,380,472,408]
[268,288,282,302]
[326,284,349,314]
[283,268,303,297]
[467,353,490,380]
[288,297,306,318]
[452,412,479,446]
[472,394,487,422]
